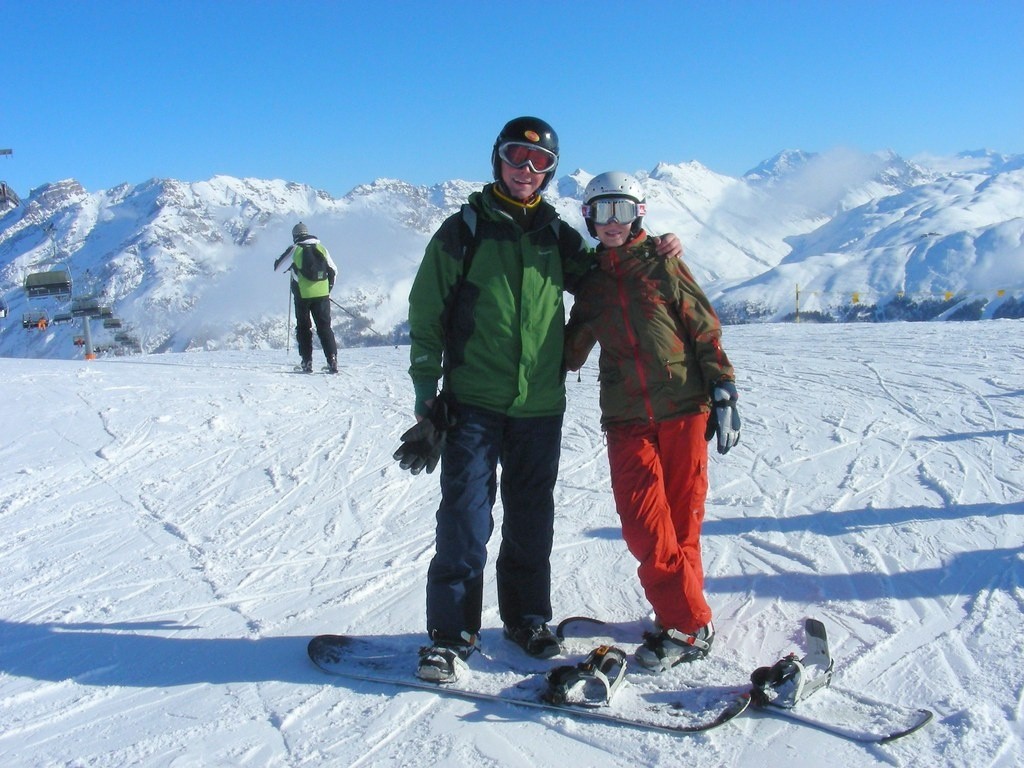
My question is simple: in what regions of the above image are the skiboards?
[294,366,338,374]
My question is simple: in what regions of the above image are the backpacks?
[293,243,327,281]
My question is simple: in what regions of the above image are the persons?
[96,347,100,353]
[408,117,684,680]
[27,316,46,331]
[78,337,83,347]
[275,222,338,373]
[563,172,740,671]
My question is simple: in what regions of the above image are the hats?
[292,222,307,238]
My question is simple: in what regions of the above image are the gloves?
[704,381,741,455]
[393,399,458,475]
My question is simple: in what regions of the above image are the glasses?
[583,202,646,224]
[497,141,558,174]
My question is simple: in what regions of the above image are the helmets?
[583,171,646,230]
[492,117,559,190]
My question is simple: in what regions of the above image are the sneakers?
[502,616,562,658]
[634,621,715,669]
[415,630,482,681]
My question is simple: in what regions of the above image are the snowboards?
[557,617,934,744]
[307,634,751,733]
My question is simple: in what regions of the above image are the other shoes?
[301,355,313,371]
[326,354,337,367]
